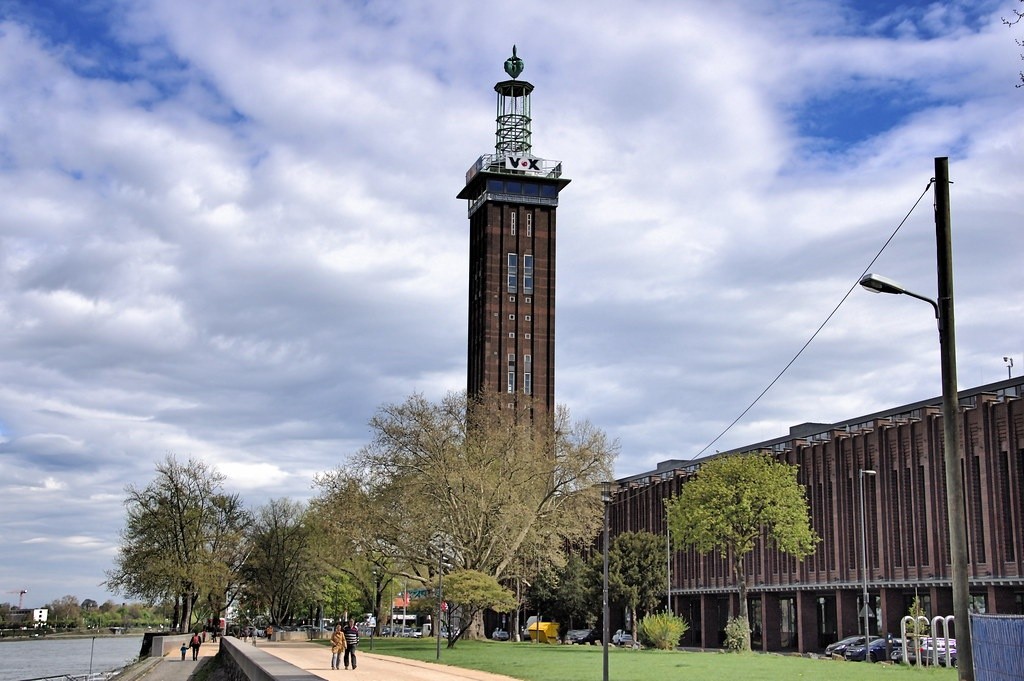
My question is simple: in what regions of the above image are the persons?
[212,628,218,643]
[189,632,201,661]
[180,643,189,661]
[202,630,206,643]
[267,626,273,641]
[242,625,258,648]
[329,619,360,670]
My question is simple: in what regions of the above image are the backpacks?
[194,636,199,645]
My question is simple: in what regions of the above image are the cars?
[568,628,600,644]
[890,634,958,670]
[823,633,880,658]
[492,630,509,640]
[241,622,460,640]
[611,628,634,646]
[845,637,912,664]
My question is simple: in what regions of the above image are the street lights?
[310,592,316,641]
[858,468,877,662]
[599,480,613,681]
[369,567,378,652]
[854,273,974,681]
[434,541,445,661]
[333,583,341,632]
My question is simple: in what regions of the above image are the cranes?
[5,590,27,610]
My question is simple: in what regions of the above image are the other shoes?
[345,666,348,670]
[352,664,357,669]
[332,666,335,670]
[337,666,339,669]
